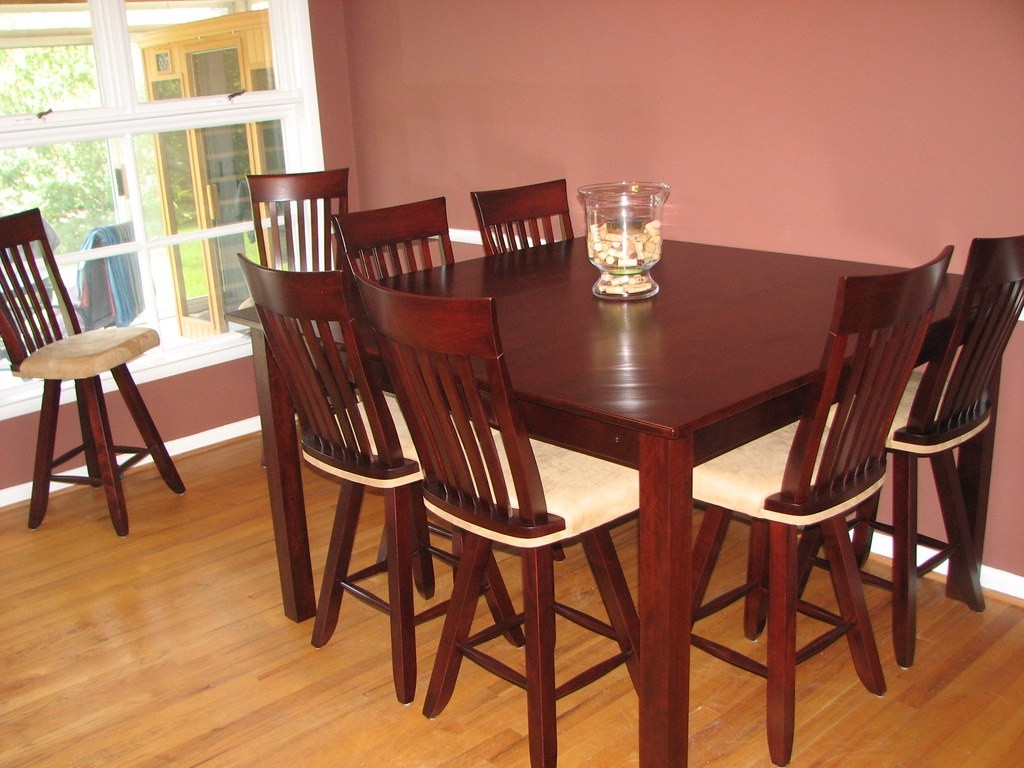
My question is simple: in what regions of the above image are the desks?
[223,236,965,767]
[0,268,53,365]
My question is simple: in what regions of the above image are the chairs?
[243,167,350,470]
[10,220,145,350]
[331,194,456,282]
[796,230,1024,670]
[689,244,955,768]
[353,268,641,768]
[236,251,528,707]
[470,179,575,257]
[0,208,186,539]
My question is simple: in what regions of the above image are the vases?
[579,181,671,300]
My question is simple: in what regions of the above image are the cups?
[578,182,670,301]
